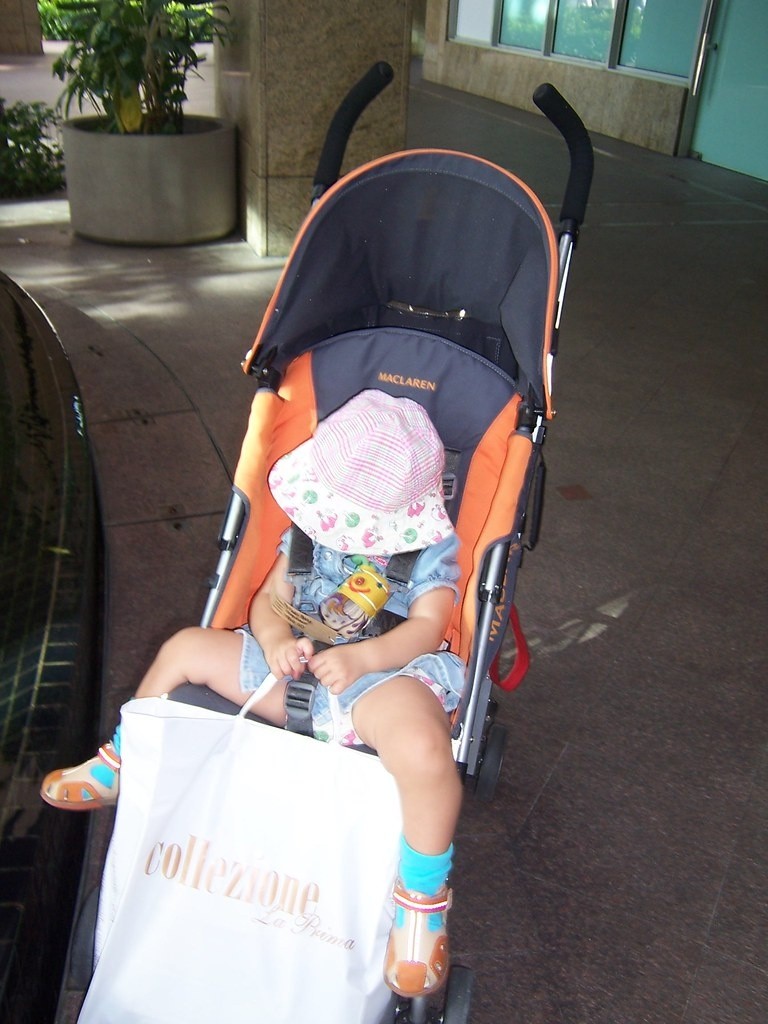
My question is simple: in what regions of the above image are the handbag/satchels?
[75,655,404,1023]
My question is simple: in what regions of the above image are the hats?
[269,391,456,555]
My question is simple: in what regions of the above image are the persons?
[41,390,465,996]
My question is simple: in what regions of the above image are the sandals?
[40,742,124,809]
[384,878,453,998]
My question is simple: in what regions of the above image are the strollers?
[160,62,594,1024]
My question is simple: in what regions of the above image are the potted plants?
[36,0,241,246]
